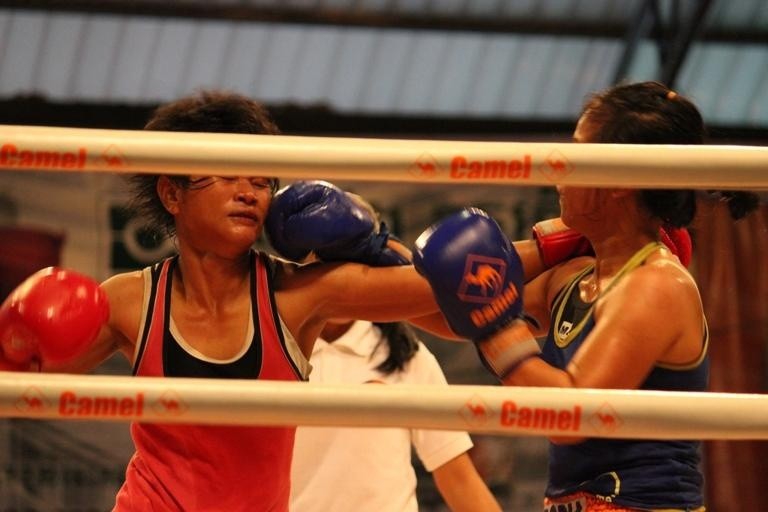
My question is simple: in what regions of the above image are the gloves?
[0,265,109,370]
[263,181,413,267]
[534,211,692,269]
[412,208,543,378]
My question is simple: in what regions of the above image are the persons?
[263,79,709,512]
[1,89,693,511]
[287,193,504,512]
